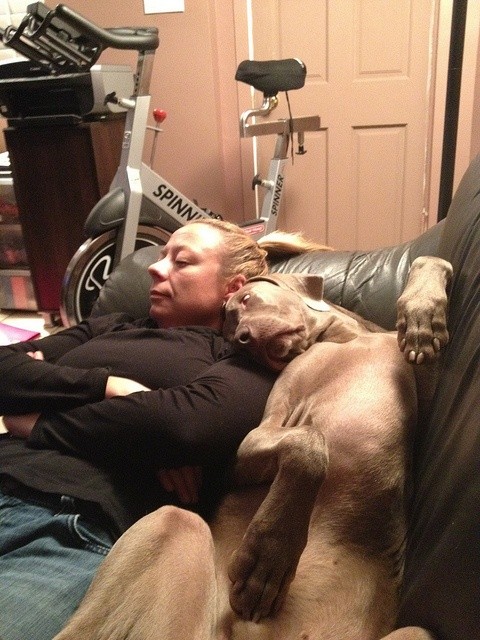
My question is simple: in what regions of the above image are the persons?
[0,218,277,640]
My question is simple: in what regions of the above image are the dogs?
[49,255,454,640]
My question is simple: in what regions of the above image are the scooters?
[1,1,321,328]
